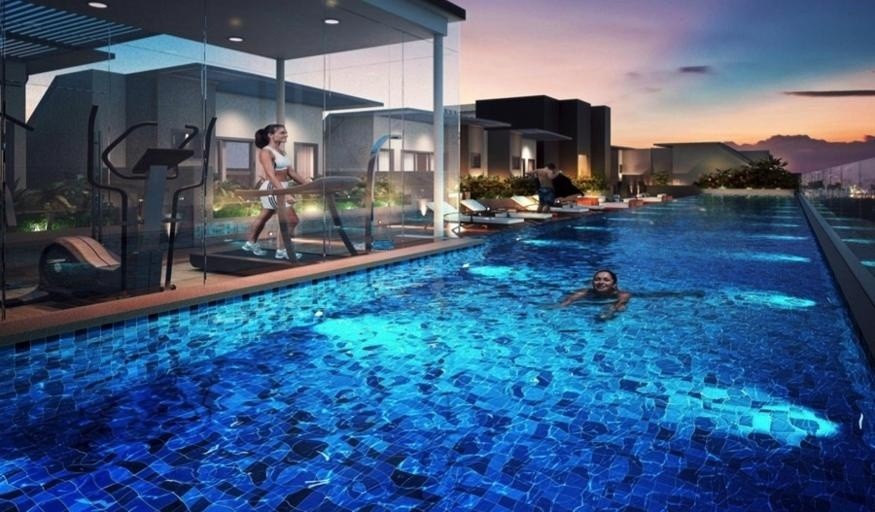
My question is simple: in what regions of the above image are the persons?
[242,123,308,261]
[542,269,632,320]
[526,162,564,214]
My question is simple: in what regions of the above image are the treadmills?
[190,175,366,277]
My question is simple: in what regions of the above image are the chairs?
[423,194,606,231]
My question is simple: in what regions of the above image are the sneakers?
[242,242,267,256]
[275,248,302,260]
